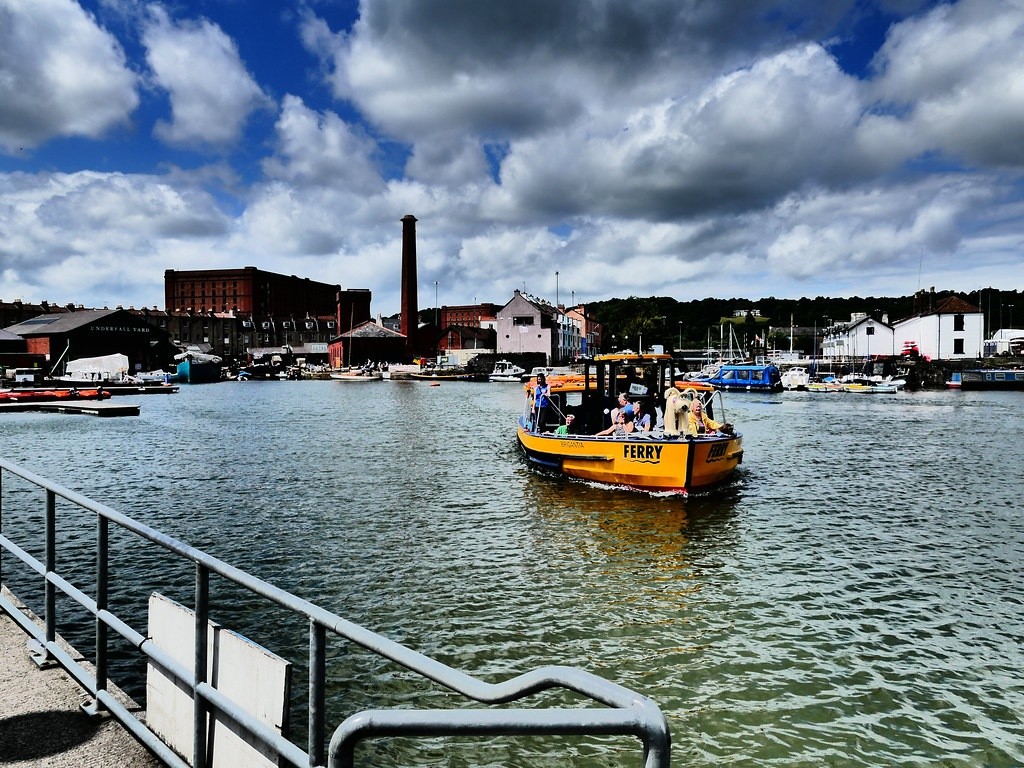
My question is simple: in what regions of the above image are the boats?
[113,369,177,384]
[780,366,907,393]
[674,361,783,392]
[411,371,485,381]
[945,368,1024,391]
[521,363,611,382]
[488,359,526,383]
[220,366,383,380]
[516,349,744,490]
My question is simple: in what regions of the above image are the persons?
[348,358,393,372]
[531,373,551,433]
[649,387,722,437]
[556,415,575,438]
[595,391,651,440]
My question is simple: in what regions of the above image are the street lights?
[823,315,828,336]
[874,309,880,321]
[571,290,575,308]
[679,320,683,349]
[638,333,642,353]
[555,271,559,307]
[435,282,437,327]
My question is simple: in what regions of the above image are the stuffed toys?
[662,387,698,437]
